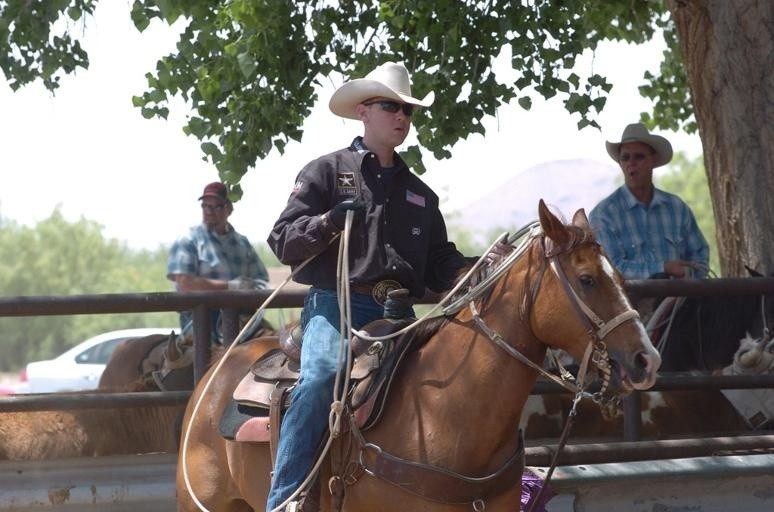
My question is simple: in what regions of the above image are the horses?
[97,327,277,390]
[519,265,774,446]
[175,199,662,512]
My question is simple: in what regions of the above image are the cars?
[17,327,183,397]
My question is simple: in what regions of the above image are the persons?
[586,122,709,302]
[266,61,510,509]
[167,182,270,344]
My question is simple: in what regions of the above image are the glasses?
[365,100,414,116]
[201,204,224,210]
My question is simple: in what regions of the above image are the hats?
[198,182,228,203]
[329,61,435,120]
[605,123,673,167]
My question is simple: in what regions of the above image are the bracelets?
[228,279,240,290]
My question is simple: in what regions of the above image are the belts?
[350,279,401,308]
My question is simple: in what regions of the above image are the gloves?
[329,198,366,231]
